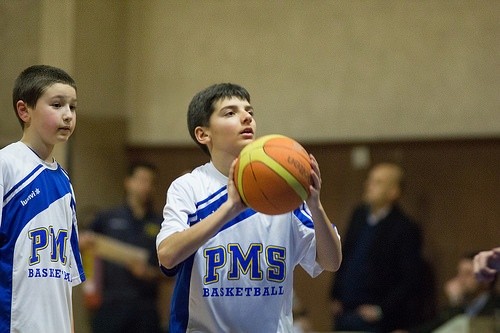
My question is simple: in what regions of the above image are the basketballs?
[233,134,314,216]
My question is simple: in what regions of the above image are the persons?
[0,65,86,333]
[79,165,500,333]
[156,82,341,333]
